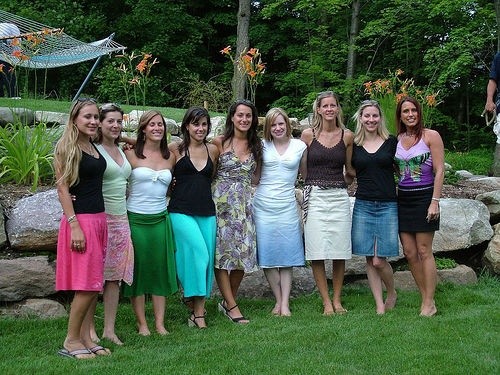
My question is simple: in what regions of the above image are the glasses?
[233,99,254,106]
[74,97,98,106]
[99,102,121,115]
[361,100,379,106]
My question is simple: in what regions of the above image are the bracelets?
[68,214,76,222]
[432,198,440,202]
[69,220,78,224]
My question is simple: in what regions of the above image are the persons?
[252,107,307,317]
[121,105,220,328]
[342,100,399,316]
[394,97,445,317]
[299,90,355,315]
[0,20,22,99]
[52,96,137,360]
[91,103,132,353]
[123,110,175,335]
[172,100,266,323]
[484,51,500,177]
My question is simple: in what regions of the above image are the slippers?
[92,346,112,357]
[57,348,97,360]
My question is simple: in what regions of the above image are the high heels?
[187,314,209,331]
[217,300,228,315]
[224,305,249,324]
[180,295,208,316]
